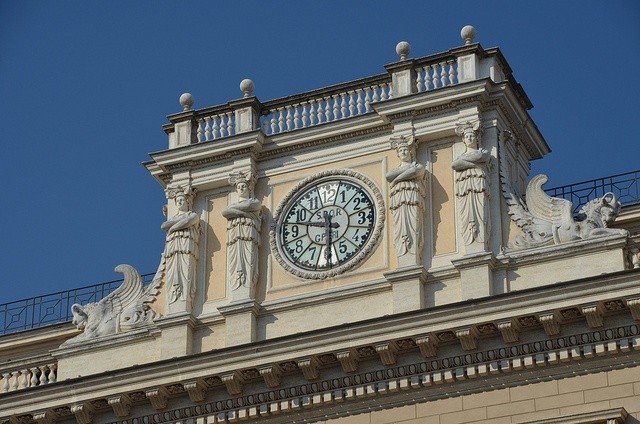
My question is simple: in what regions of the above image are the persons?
[223,170,264,302]
[160,188,202,315]
[386,136,428,269]
[451,123,492,254]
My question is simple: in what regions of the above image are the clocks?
[274,175,377,274]
